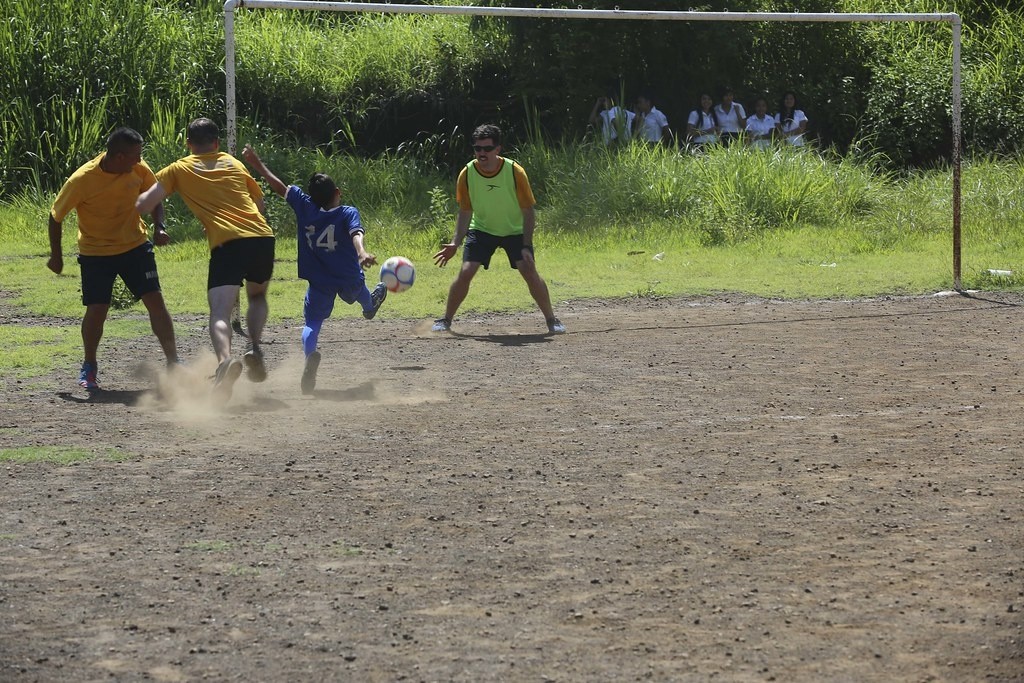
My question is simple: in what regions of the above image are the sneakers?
[79,362,101,389]
[243,350,266,382]
[362,282,388,319]
[432,317,452,331]
[546,317,567,334]
[301,351,321,394]
[208,358,243,406]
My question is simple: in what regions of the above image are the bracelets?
[521,245,533,252]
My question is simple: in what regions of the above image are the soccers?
[379,256,416,293]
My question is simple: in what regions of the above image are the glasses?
[471,144,498,152]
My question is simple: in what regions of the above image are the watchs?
[154,221,167,230]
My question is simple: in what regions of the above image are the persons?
[46,127,196,390]
[136,116,274,405]
[241,144,388,393]
[432,124,564,333]
[599,85,808,152]
